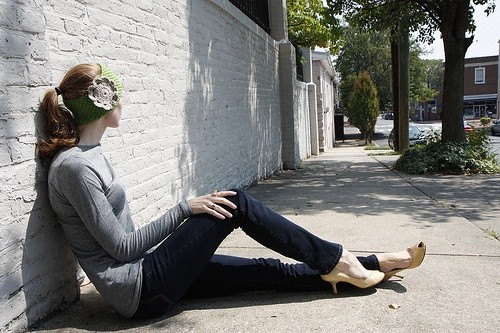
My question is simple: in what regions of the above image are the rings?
[211,189,218,196]
[212,203,217,209]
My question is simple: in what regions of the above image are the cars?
[491,119,500,135]
[387,124,428,153]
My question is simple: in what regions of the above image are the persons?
[38,61,427,318]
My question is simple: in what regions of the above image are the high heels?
[381,242,426,283]
[321,267,385,294]
